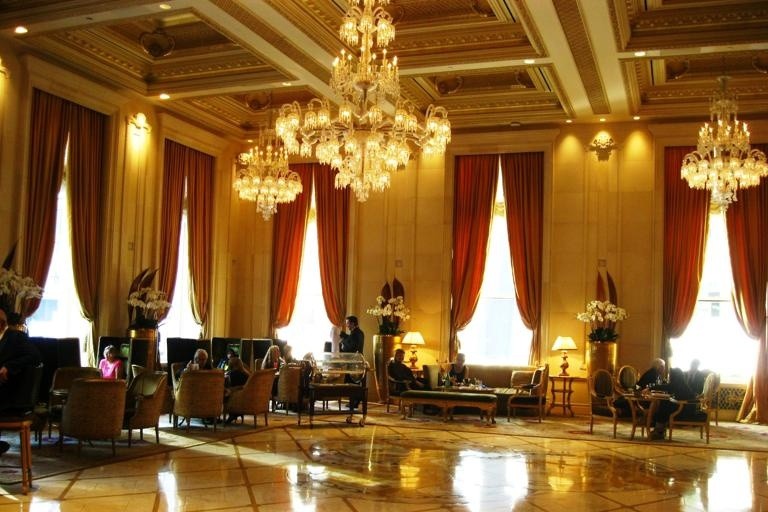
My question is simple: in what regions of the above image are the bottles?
[444,371,450,387]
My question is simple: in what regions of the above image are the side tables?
[548,376,583,418]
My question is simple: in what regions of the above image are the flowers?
[0,266,45,325]
[126,288,171,329]
[367,295,410,335]
[576,299,628,342]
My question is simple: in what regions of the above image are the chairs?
[588,365,721,444]
[384,360,420,416]
[506,363,550,423]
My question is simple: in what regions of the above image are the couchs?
[422,364,541,417]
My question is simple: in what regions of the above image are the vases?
[130,329,157,373]
[372,334,402,404]
[589,341,617,414]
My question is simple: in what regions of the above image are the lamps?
[551,335,577,375]
[274,1,451,204]
[230,91,302,223]
[681,54,768,214]
[401,331,425,369]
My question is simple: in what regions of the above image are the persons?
[224,358,251,424]
[339,316,365,406]
[98,344,123,380]
[217,346,240,369]
[651,369,696,440]
[636,358,667,389]
[261,345,286,369]
[389,348,435,414]
[442,352,470,385]
[177,348,211,380]
[684,358,704,395]
[0,310,41,457]
[283,346,295,363]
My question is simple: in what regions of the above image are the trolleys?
[295,351,370,430]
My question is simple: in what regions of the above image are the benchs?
[399,391,497,427]
